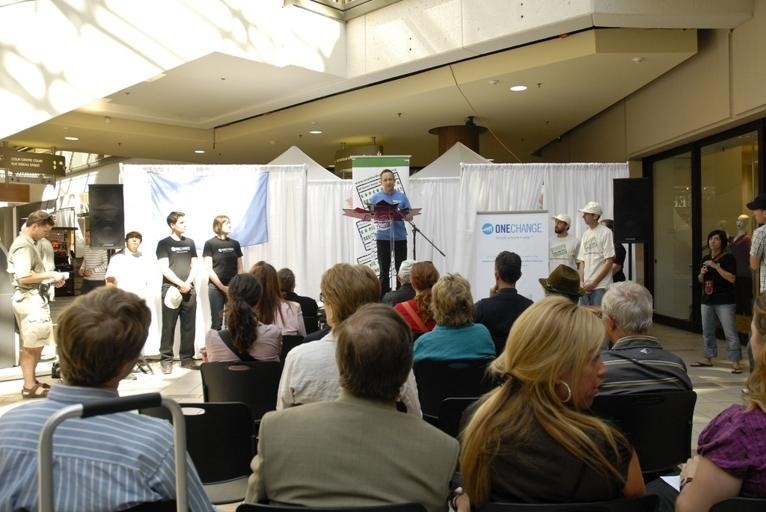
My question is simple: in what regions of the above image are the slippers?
[733,368,742,373]
[690,361,713,367]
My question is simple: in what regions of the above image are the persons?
[1,251,766,511]
[7,208,243,398]
[368,167,412,294]
[548,201,626,308]
[690,193,766,376]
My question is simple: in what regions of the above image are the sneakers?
[162,362,172,373]
[181,359,201,369]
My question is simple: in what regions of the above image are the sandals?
[21,382,51,399]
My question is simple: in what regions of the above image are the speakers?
[89,184,124,249]
[613,178,647,244]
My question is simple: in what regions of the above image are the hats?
[164,286,184,309]
[579,202,604,216]
[538,264,586,296]
[746,192,766,210]
[552,214,571,226]
